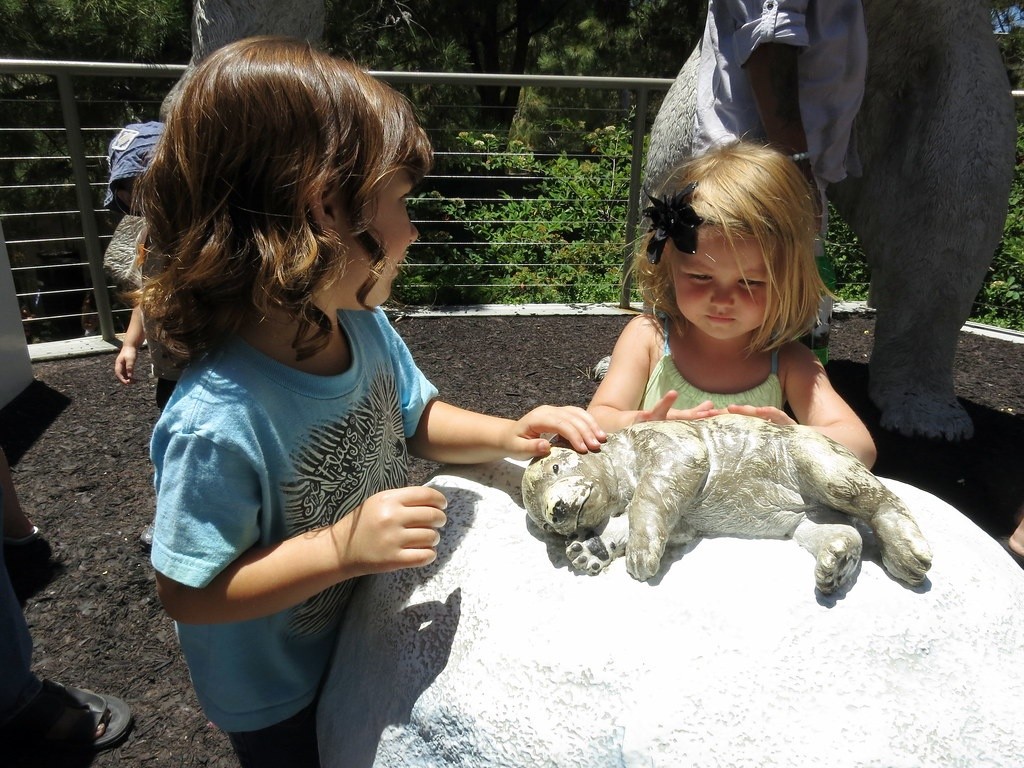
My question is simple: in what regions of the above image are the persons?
[102,119,163,384]
[0,443,131,768]
[585,135,878,474]
[125,37,608,768]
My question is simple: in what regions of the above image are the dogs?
[521,414,932,597]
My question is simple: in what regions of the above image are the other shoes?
[3,528,52,574]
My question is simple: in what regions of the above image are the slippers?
[3,679,133,753]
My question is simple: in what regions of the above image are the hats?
[102,121,166,215]
